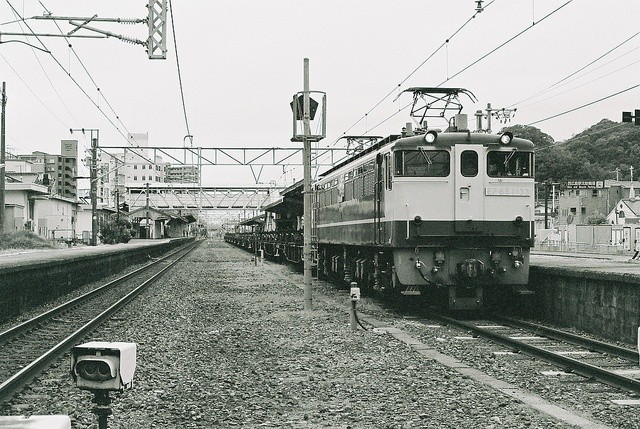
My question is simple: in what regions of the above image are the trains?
[224,131,534,311]
